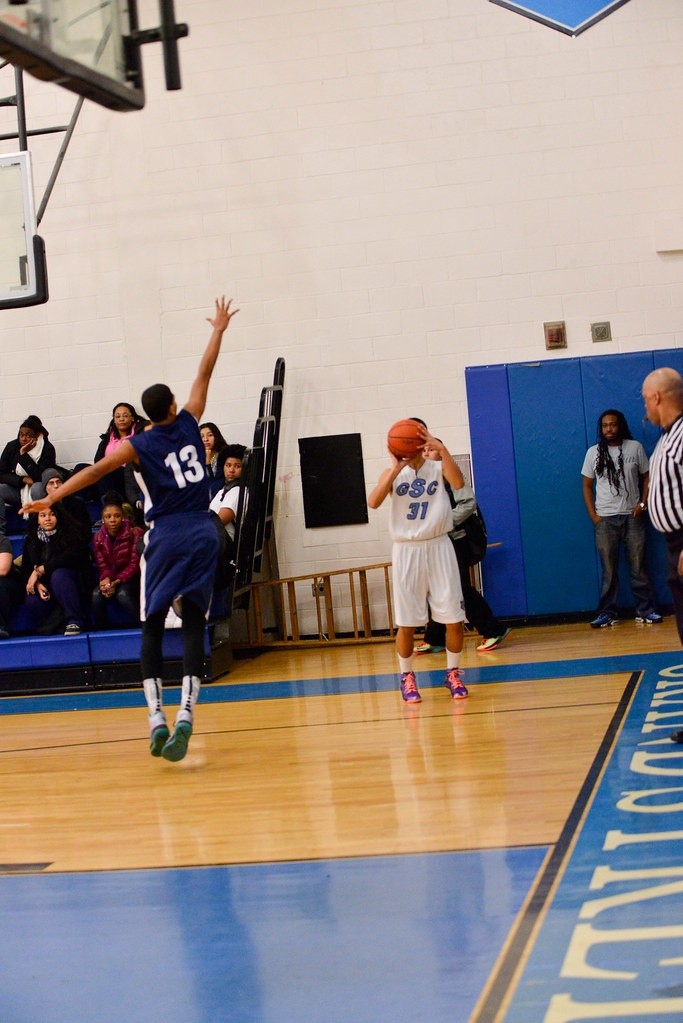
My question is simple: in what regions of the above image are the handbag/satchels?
[443,476,487,565]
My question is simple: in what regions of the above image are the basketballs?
[387,419,427,459]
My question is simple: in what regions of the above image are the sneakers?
[413,640,445,655]
[634,610,663,625]
[400,671,422,702]
[477,628,511,650]
[148,712,193,762]
[445,668,470,699]
[64,623,82,637]
[590,614,623,628]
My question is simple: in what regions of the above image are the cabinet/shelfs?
[461,346,683,627]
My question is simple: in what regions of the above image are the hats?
[21,415,43,432]
[42,468,64,490]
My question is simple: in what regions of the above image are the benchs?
[0,475,231,692]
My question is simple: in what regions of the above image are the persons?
[413,438,511,655]
[642,368,683,743]
[368,417,469,703]
[580,409,663,628]
[0,403,250,639]
[17,297,240,763]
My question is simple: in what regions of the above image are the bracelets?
[639,502,647,510]
[34,565,43,580]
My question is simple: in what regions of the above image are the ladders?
[230,542,503,648]
